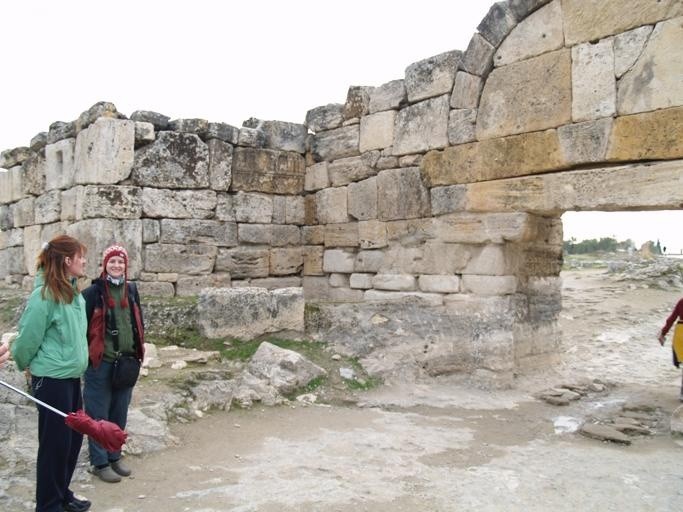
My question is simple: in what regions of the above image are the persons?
[8,233,92,512]
[656,297,683,403]
[78,243,145,483]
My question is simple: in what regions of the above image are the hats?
[102,243,128,308]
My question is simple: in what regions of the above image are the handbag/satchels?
[112,352,142,388]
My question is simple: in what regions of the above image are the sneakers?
[111,459,130,475]
[92,465,120,482]
[67,495,90,512]
